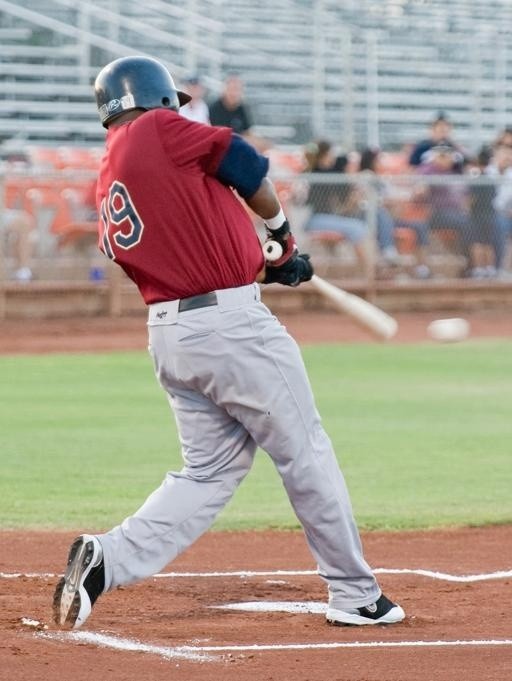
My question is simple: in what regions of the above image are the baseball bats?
[263,240,397,341]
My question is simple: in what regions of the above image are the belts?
[179,292,218,313]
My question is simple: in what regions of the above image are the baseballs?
[429,316,469,344]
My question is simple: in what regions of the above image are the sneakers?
[326,594,406,626]
[51,533,105,631]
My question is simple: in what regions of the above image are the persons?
[295,143,432,281]
[180,72,283,177]
[50,53,408,631]
[409,118,512,277]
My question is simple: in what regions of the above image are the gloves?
[261,217,312,287]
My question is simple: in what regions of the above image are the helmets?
[94,55,192,129]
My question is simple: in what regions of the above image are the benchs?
[0,0,511,148]
